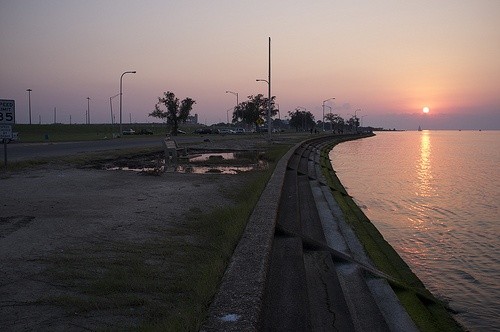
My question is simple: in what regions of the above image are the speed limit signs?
[0,99,16,125]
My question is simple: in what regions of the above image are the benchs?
[162,139,188,159]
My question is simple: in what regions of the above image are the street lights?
[256,36,271,147]
[26,89,33,125]
[322,97,335,132]
[109,93,122,124]
[226,90,239,135]
[322,104,333,132]
[119,70,137,137]
[86,97,90,124]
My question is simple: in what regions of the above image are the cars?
[135,128,154,136]
[122,128,135,135]
[0,132,18,144]
[192,129,245,135]
[170,130,186,135]
